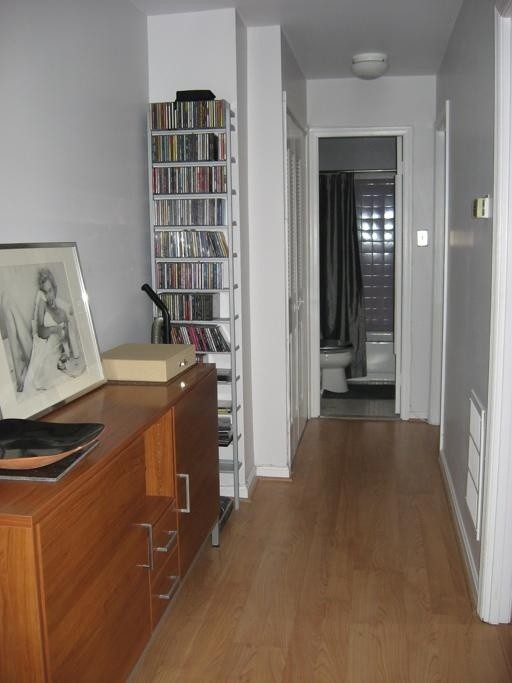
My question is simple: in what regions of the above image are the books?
[146,98,235,447]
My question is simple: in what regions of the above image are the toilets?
[320,341,355,394]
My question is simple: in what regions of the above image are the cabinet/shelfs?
[0,364,219,683]
[147,100,242,547]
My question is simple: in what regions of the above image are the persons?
[21,265,74,397]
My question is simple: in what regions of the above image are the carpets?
[322,382,396,400]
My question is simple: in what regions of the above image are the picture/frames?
[0,242,108,419]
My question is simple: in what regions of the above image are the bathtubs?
[345,331,396,386]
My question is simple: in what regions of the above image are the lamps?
[351,53,388,79]
